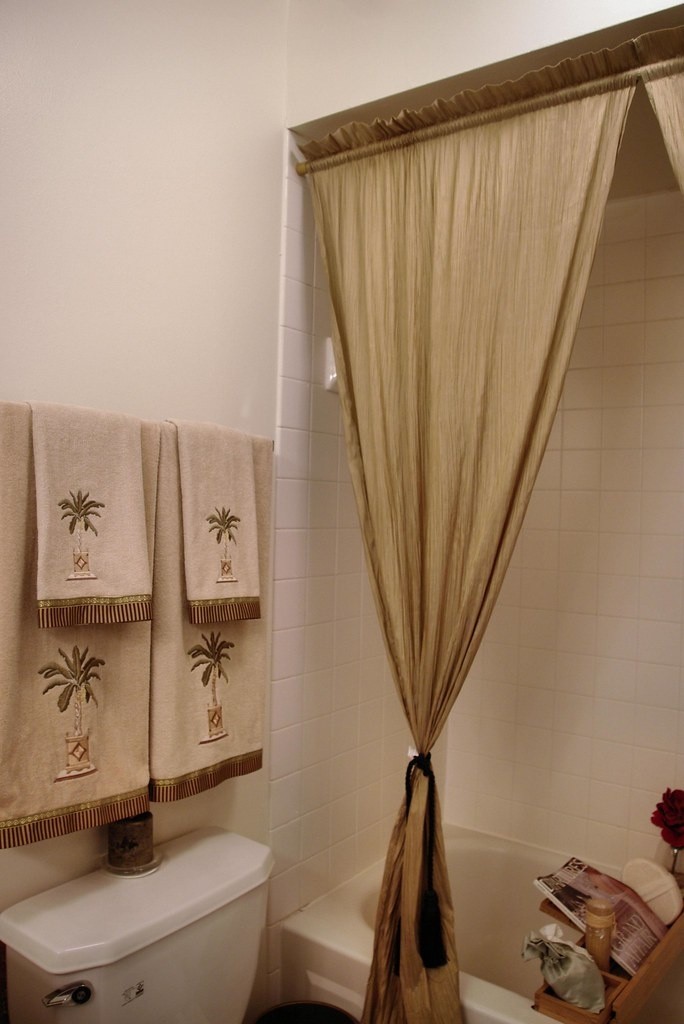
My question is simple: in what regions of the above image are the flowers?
[651,786,684,875]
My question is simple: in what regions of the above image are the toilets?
[0,824,277,1024]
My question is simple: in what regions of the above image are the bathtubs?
[279,818,624,1024]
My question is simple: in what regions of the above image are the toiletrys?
[584,898,616,973]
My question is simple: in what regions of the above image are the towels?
[0,398,275,851]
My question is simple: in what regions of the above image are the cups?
[585,898,615,972]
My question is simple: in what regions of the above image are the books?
[534,855,665,977]
[538,898,582,934]
